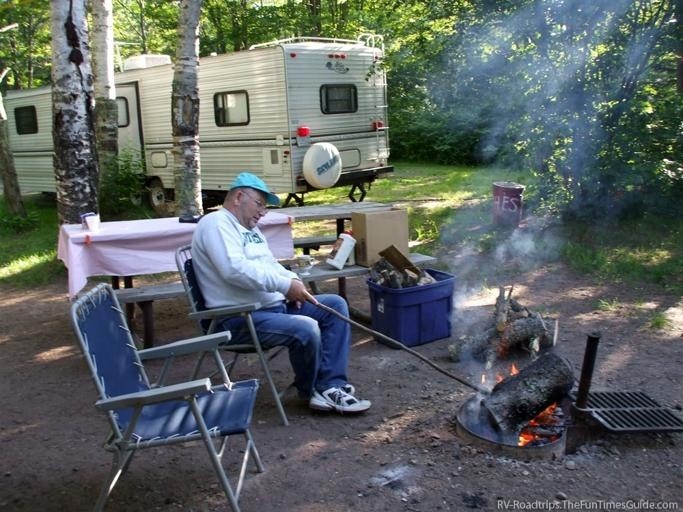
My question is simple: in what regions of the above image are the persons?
[189,170,373,416]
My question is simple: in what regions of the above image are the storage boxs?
[352,207,457,349]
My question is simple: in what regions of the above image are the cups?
[296,255,315,277]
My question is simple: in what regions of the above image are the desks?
[60,201,395,362]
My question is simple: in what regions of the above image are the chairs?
[71,244,302,511]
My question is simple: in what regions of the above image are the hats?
[230,172,280,206]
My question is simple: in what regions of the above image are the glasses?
[240,189,271,214]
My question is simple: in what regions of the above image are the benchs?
[75,236,448,363]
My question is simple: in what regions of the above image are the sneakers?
[304,383,371,412]
